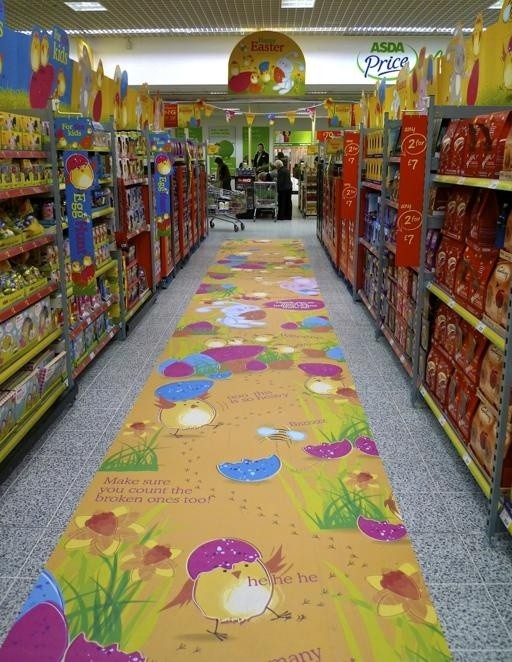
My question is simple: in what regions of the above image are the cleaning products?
[253,181,278,223]
[206,183,248,232]
[206,182,219,216]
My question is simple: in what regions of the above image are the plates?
[298,164,316,217]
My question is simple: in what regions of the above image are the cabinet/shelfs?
[52,115,127,380]
[374,106,433,408]
[298,164,316,217]
[2,111,79,479]
[113,126,159,342]
[412,106,512,541]
[148,123,208,292]
[315,120,365,303]
[353,111,388,342]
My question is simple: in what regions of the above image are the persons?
[271,160,293,221]
[277,152,288,169]
[253,142,270,173]
[214,157,232,190]
